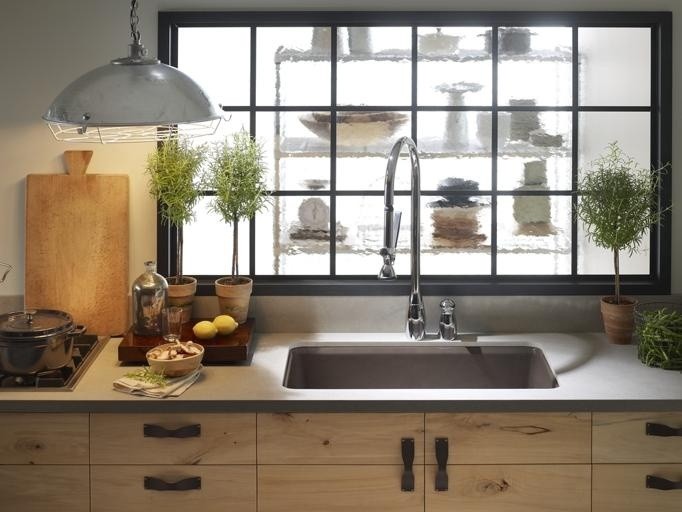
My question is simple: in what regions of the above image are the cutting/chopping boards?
[24,150,129,337]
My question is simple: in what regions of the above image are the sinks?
[281,342,560,389]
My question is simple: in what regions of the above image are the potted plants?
[201,123,275,324]
[143,136,213,324]
[570,137,675,345]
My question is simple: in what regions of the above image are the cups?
[161,308,183,342]
[310,28,375,55]
[478,110,510,152]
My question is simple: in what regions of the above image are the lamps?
[42,0,233,143]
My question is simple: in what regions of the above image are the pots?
[418,27,467,57]
[477,28,537,55]
[1,307,88,376]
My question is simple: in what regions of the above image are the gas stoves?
[1,331,111,392]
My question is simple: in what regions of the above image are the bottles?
[130,260,169,336]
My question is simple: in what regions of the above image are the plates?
[293,221,344,236]
[297,197,331,229]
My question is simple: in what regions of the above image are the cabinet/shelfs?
[274,44,589,275]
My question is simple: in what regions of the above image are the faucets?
[376,135,426,341]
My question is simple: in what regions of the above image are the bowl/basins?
[313,109,394,121]
[303,179,330,188]
[146,342,205,376]
[437,178,480,200]
[300,117,411,146]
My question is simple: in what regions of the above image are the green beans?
[637,308,682,367]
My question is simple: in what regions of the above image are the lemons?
[192,320,218,340]
[213,315,239,336]
